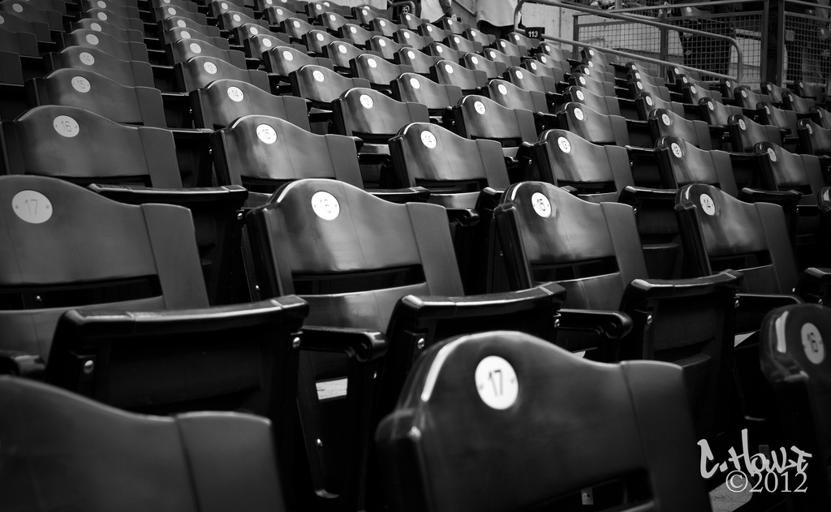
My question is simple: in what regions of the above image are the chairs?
[1,1,831,511]
